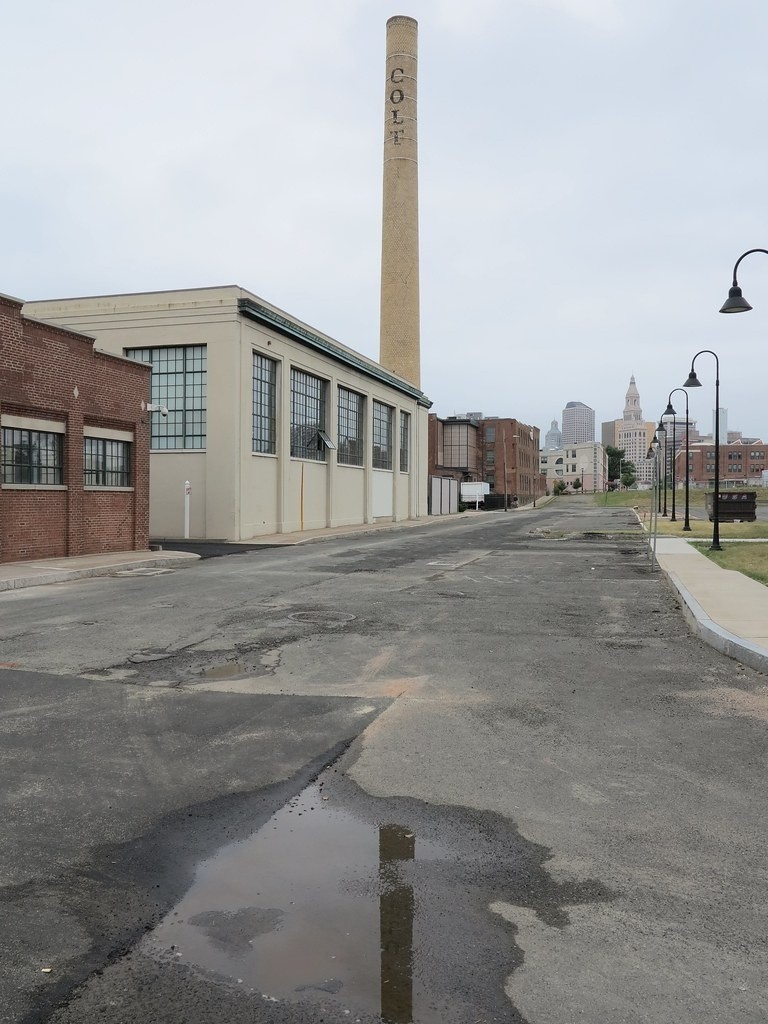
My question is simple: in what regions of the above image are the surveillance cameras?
[161,408,168,416]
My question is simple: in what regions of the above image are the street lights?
[683,349,723,551]
[619,458,625,493]
[651,436,667,517]
[663,387,693,531]
[502,427,521,512]
[646,449,657,513]
[655,411,676,522]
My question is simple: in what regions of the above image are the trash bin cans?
[483,493,512,509]
[704,491,758,523]
